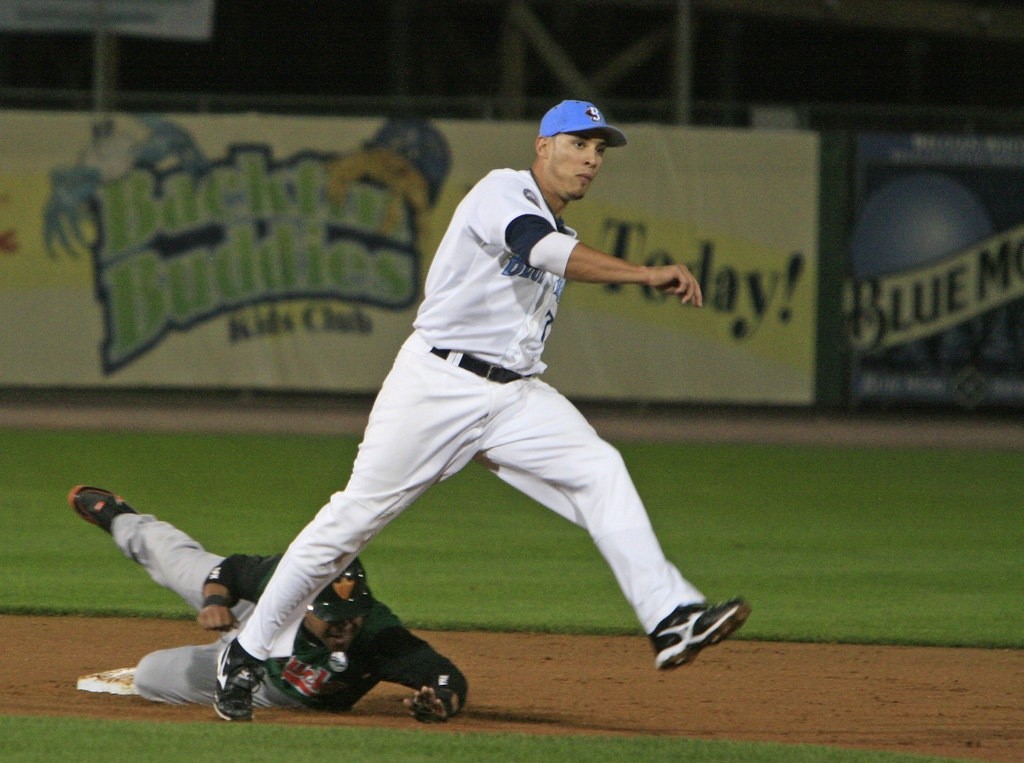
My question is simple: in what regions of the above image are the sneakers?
[649,596,750,672]
[70,486,137,533]
[213,637,269,722]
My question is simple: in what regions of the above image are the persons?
[67,485,468,724]
[213,101,751,725]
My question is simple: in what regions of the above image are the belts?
[431,347,530,383]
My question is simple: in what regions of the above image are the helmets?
[311,556,373,621]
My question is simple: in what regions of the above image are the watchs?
[203,594,225,607]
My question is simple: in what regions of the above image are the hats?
[539,100,627,148]
